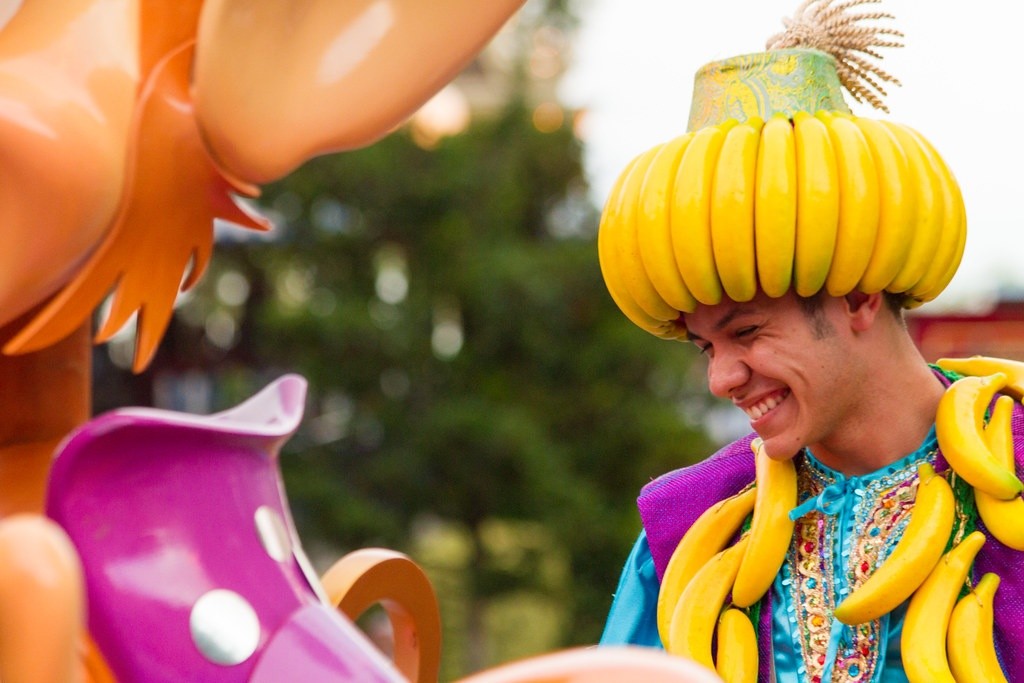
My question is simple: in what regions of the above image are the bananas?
[599,111,967,345]
[656,356,1024,683]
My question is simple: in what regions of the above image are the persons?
[597,0,1024,683]
